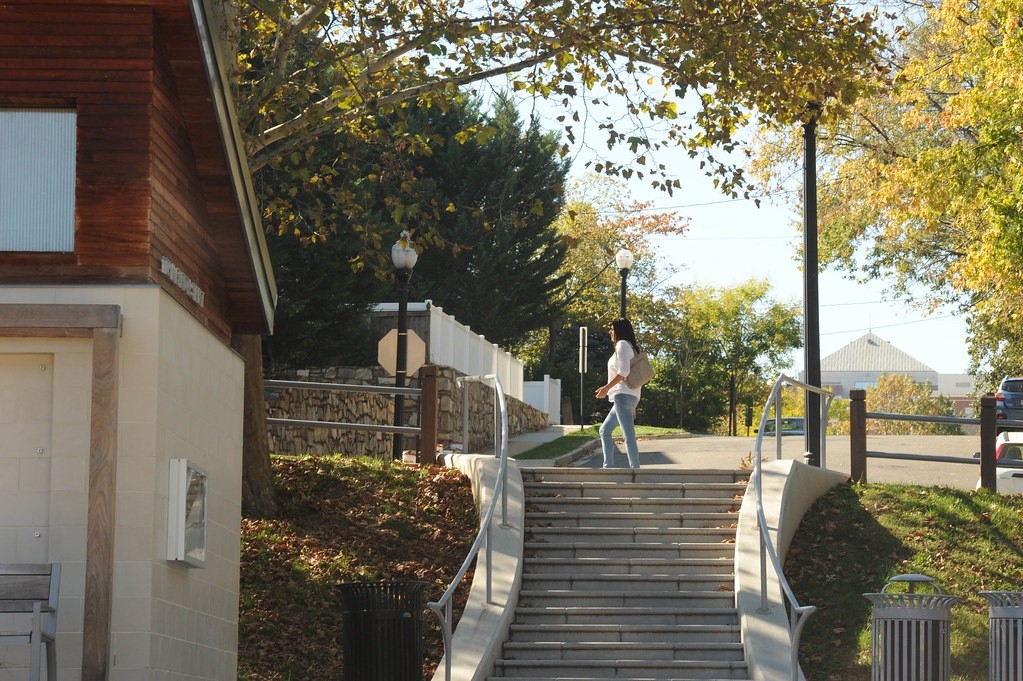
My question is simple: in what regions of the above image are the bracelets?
[604,386,609,390]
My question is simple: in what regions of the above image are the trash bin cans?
[977,590,1023,681]
[861,574,963,681]
[333,581,429,681]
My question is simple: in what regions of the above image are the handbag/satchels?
[614,340,656,389]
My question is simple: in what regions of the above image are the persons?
[595,318,642,469]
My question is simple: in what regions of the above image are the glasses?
[609,328,615,331]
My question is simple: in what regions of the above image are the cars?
[972,431,1023,495]
[756,418,804,436]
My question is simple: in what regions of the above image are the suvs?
[994,375,1023,434]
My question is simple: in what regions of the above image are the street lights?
[617,249,633,317]
[389,229,419,458]
